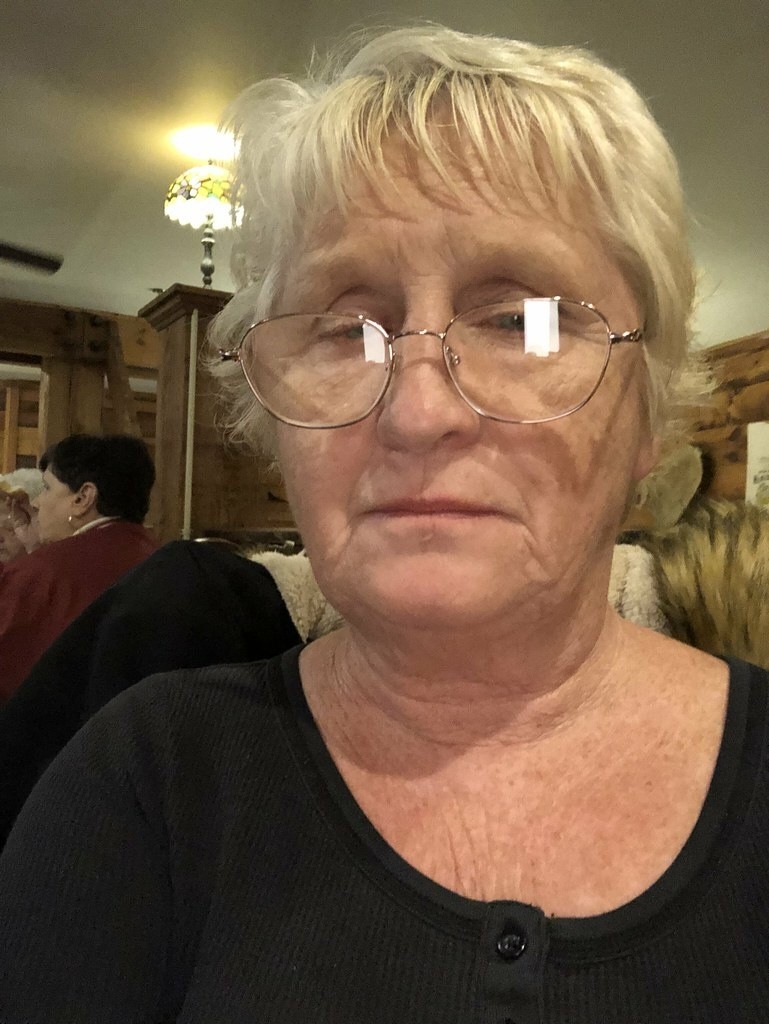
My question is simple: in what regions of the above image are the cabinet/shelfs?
[138,283,304,555]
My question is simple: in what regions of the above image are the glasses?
[216,293,650,432]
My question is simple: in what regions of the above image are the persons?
[0,24,769,1024]
[0,433,162,707]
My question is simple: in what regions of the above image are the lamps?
[164,158,247,288]
